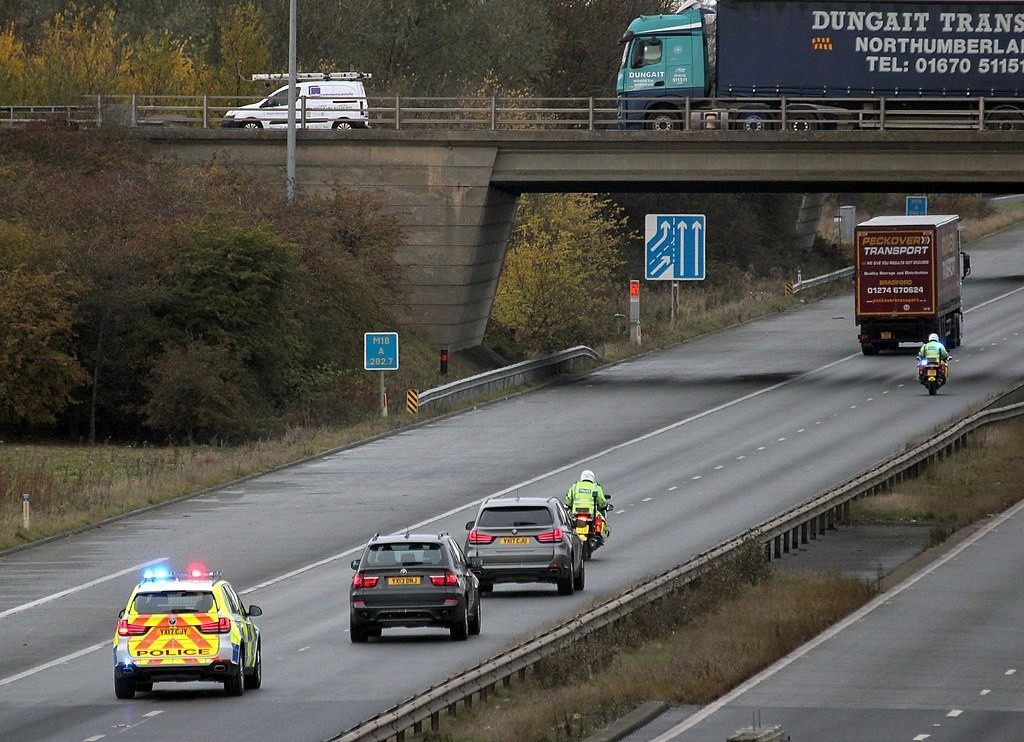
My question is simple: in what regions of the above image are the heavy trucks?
[613,1,1024,133]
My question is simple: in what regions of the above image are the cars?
[110,562,263,701]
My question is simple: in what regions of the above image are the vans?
[222,70,375,130]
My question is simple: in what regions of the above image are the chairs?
[143,602,161,614]
[400,554,415,564]
[423,551,441,565]
[151,593,169,604]
[194,601,211,613]
[378,552,397,564]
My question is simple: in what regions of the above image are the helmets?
[581,470,595,483]
[928,334,939,342]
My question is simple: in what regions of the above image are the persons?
[918,333,949,377]
[565,469,608,540]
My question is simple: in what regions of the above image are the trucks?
[853,213,972,356]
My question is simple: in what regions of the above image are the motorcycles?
[914,353,953,396]
[562,492,613,561]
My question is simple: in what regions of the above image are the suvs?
[462,496,588,596]
[348,532,484,644]
